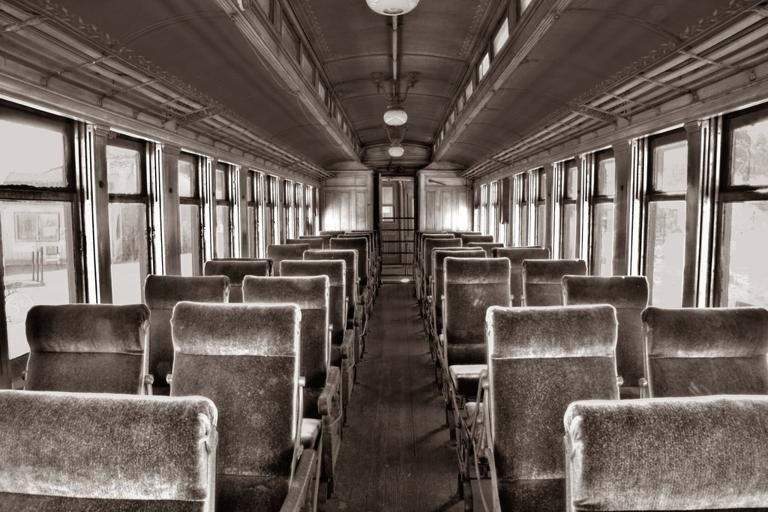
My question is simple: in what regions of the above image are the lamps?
[365,0,421,157]
